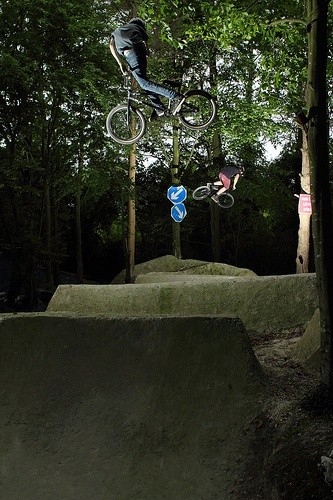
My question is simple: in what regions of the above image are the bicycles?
[105,65,219,145]
[191,182,235,209]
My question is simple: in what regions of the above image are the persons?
[110,18,185,122]
[207,165,245,203]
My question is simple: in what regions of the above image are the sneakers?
[148,105,167,121]
[172,94,185,115]
[210,194,220,203]
[212,182,219,190]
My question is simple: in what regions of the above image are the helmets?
[130,18,147,30]
[241,167,247,175]
